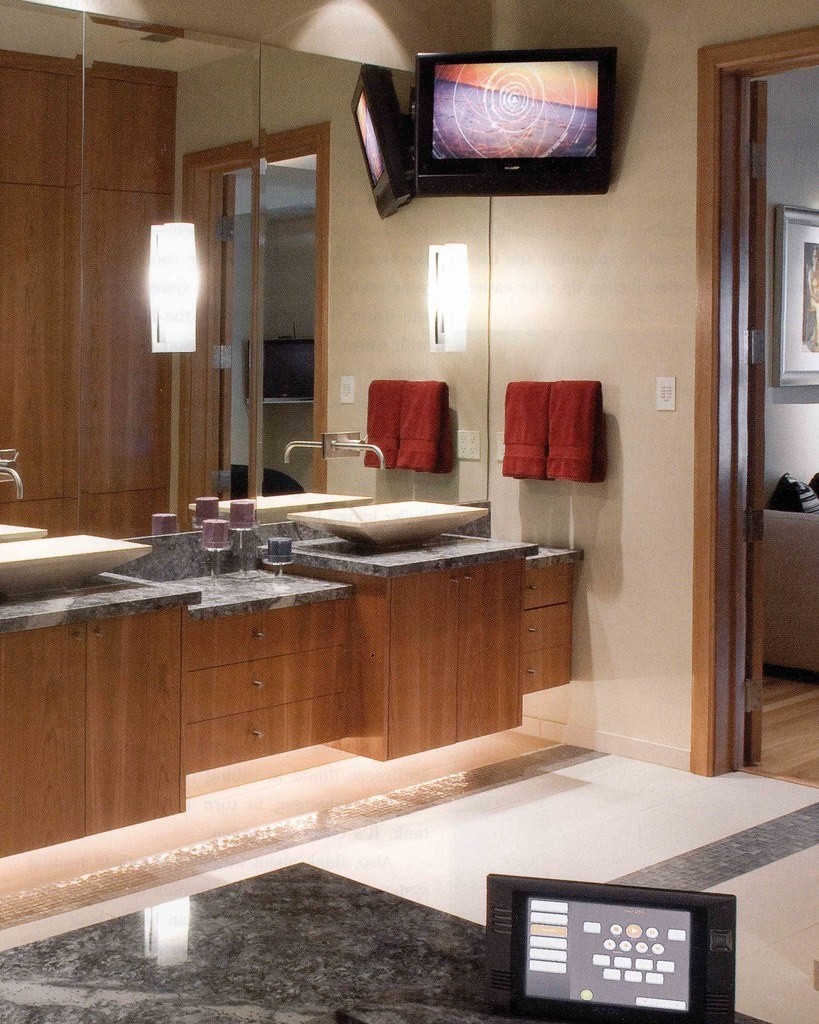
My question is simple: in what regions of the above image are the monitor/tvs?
[414,45,618,198]
[351,63,414,217]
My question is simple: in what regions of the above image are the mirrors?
[0,0,491,548]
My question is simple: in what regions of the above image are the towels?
[363,379,454,474]
[500,380,608,484]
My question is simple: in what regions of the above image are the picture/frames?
[771,204,819,387]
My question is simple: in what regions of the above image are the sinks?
[1,524,48,543]
[188,492,375,525]
[285,500,489,547]
[0,534,153,591]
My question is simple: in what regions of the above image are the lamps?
[149,221,200,353]
[428,242,469,352]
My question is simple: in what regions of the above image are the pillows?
[767,475,819,515]
[808,472,819,500]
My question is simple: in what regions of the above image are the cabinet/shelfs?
[521,561,570,693]
[184,597,352,776]
[0,610,187,858]
[270,556,522,761]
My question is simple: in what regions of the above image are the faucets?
[283,440,322,464]
[334,442,387,471]
[0,467,24,500]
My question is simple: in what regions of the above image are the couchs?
[761,402,819,683]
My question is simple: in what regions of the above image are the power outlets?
[453,429,481,460]
[496,431,505,461]
